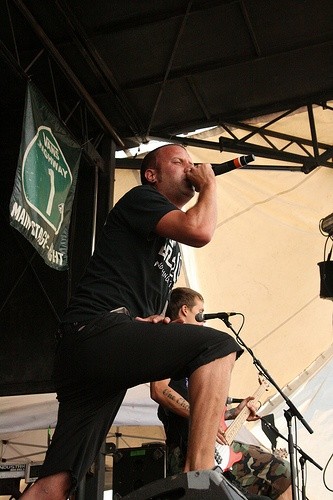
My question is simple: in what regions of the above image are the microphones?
[213,153,255,175]
[195,312,236,322]
[226,397,244,404]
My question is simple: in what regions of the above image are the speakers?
[113,446,167,500]
[117,469,248,500]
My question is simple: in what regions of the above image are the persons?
[18,144,244,500]
[149,287,300,500]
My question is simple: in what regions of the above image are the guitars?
[214,372,274,472]
